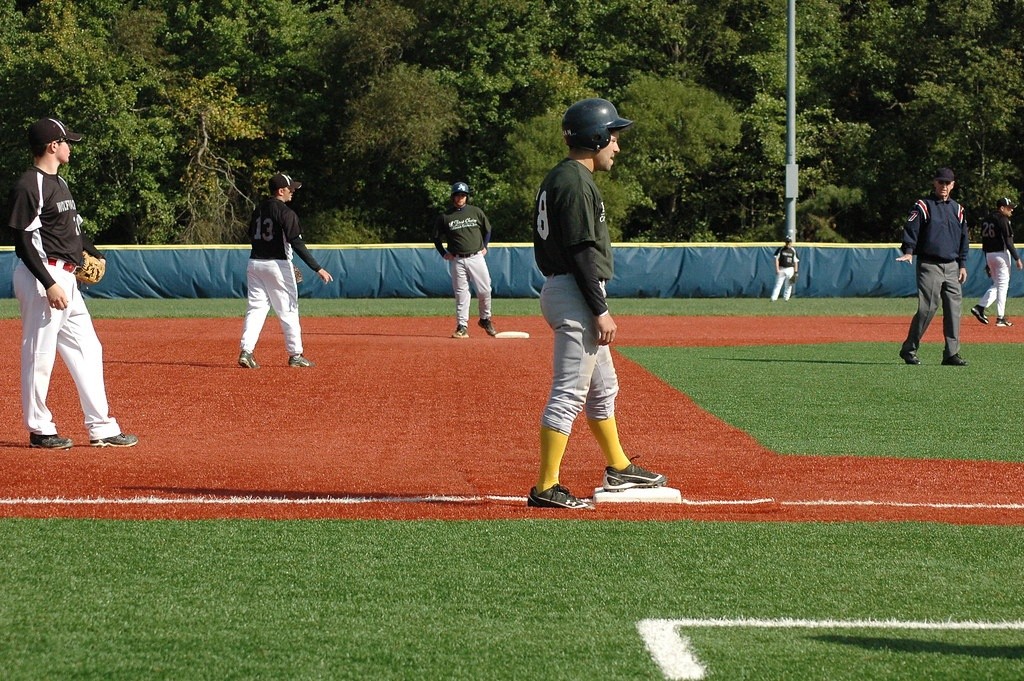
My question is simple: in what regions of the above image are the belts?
[47,258,79,277]
[451,251,478,257]
[553,272,603,283]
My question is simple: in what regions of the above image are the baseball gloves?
[77,250,106,284]
[790,272,798,283]
[293,264,303,284]
[984,264,991,279]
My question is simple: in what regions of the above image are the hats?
[996,198,1018,209]
[784,235,792,241]
[935,167,954,182]
[28,118,83,148]
[268,173,302,189]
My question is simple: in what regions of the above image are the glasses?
[936,180,951,186]
[55,138,69,144]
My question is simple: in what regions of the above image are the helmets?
[451,181,469,195]
[562,97,633,152]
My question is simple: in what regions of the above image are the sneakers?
[239,351,260,368]
[452,327,469,338]
[29,433,73,451]
[941,350,969,366]
[971,305,989,324]
[603,455,668,493]
[288,354,314,367]
[478,318,496,336]
[90,433,145,443]
[527,483,588,509]
[899,350,921,365]
[996,317,1013,327]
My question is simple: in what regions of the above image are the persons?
[770,235,800,304]
[896,168,971,365]
[238,173,333,368]
[527,98,668,511]
[970,197,1023,326]
[433,182,496,338]
[8,118,138,451]
